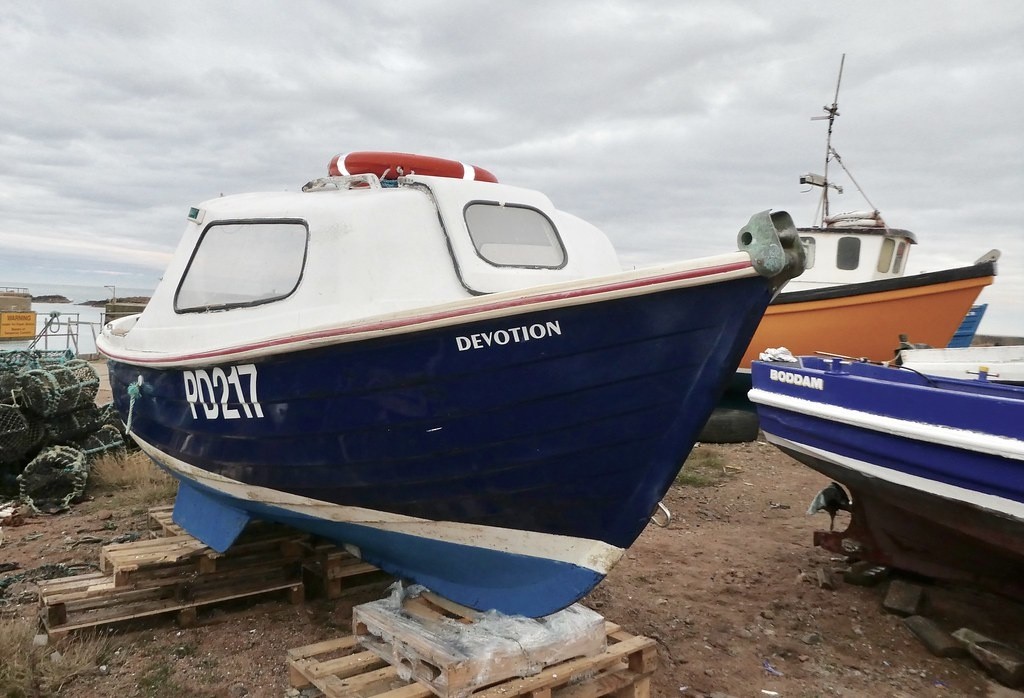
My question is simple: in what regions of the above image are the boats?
[746,333,1024,612]
[721,51,1004,399]
[93,145,820,622]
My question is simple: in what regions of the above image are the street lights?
[103,285,116,303]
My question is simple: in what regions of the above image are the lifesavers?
[328,149,499,188]
